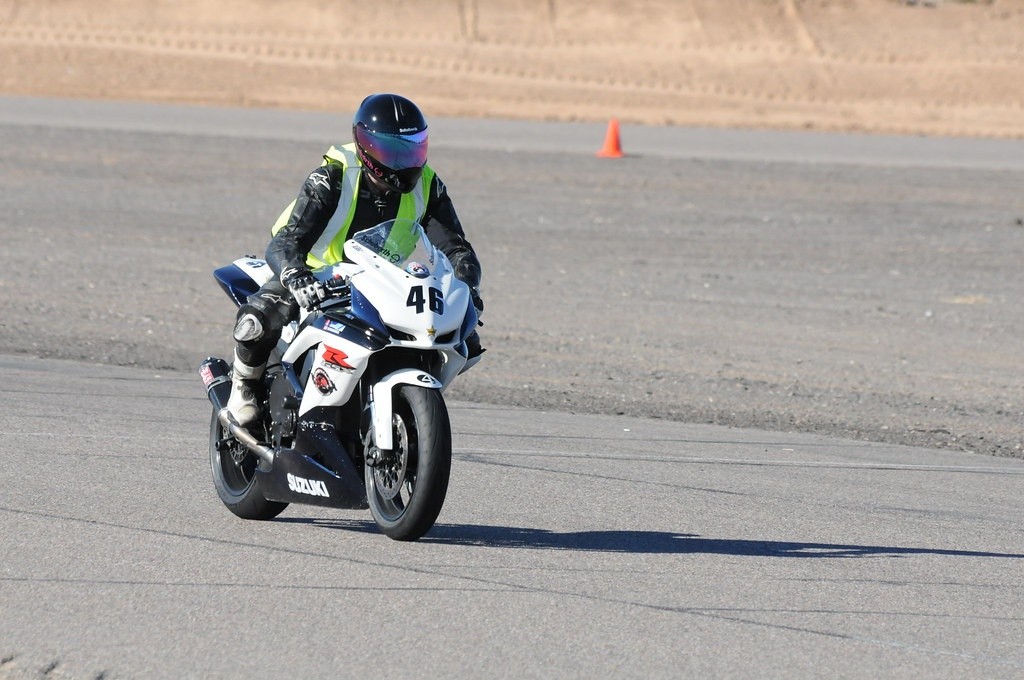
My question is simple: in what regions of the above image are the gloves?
[470,289,484,322]
[282,269,326,310]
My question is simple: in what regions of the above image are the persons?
[225,93,484,427]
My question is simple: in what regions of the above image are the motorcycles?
[200,218,488,542]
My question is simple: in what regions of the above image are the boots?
[227,345,268,428]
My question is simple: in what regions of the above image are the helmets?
[352,93,429,194]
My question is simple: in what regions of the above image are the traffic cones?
[593,118,624,159]
